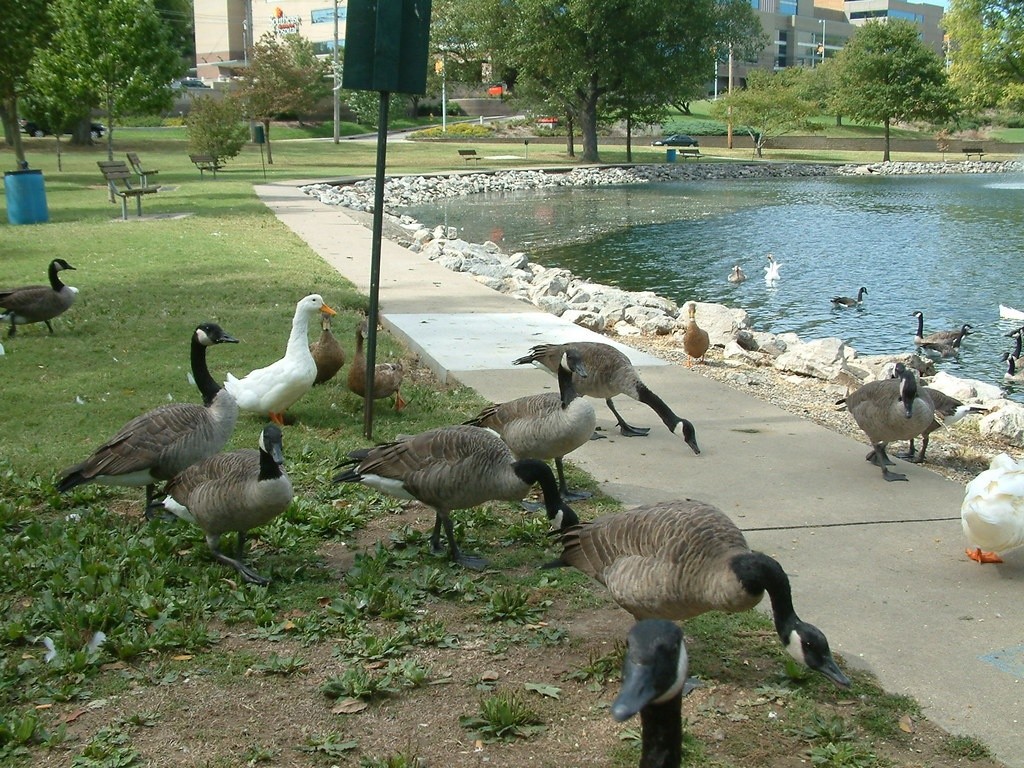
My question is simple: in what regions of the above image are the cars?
[651,133,700,148]
[180,79,211,89]
[17,115,107,141]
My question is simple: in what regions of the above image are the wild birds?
[546,498,852,689]
[148,425,294,582]
[347,321,415,411]
[463,349,598,500]
[831,287,869,309]
[0,258,79,337]
[331,424,579,563]
[53,323,240,514]
[684,304,708,363]
[833,360,964,481]
[728,266,746,282]
[906,311,973,345]
[511,342,700,455]
[916,325,973,363]
[999,304,1024,380]
[761,255,782,281]
[309,311,345,385]
[612,620,689,768]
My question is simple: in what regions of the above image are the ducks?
[960,452,1024,564]
[224,294,335,428]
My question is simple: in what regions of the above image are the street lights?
[819,19,826,64]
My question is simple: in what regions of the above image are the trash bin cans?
[6,169,49,226]
[667,149,676,162]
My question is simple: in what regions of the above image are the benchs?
[680,149,704,161]
[98,161,160,219]
[126,153,158,188]
[189,153,224,179]
[962,149,985,161]
[458,149,482,166]
